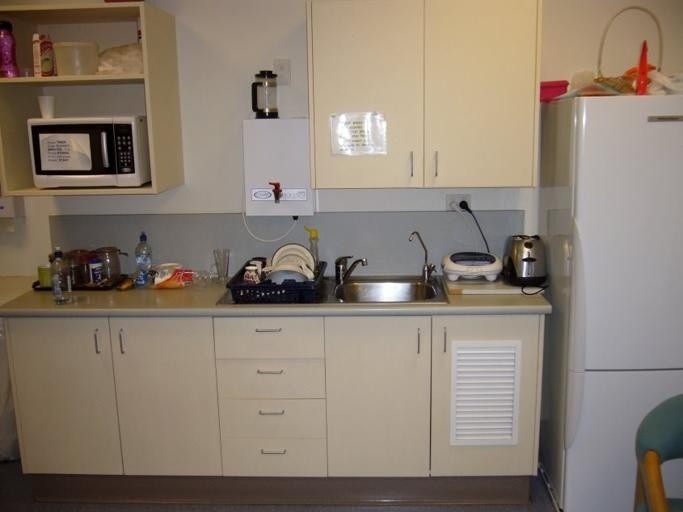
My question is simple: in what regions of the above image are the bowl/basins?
[51,42,100,75]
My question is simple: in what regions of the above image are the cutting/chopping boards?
[443,278,543,294]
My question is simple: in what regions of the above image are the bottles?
[0,20,19,78]
[134,231,151,287]
[49,244,73,306]
[243,266,259,284]
[88,258,103,285]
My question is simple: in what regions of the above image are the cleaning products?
[304,225,319,263]
[136,229,152,284]
[88,252,104,286]
[52,247,72,305]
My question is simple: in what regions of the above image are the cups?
[213,248,231,284]
[249,69,279,119]
[35,95,55,120]
[38,267,52,287]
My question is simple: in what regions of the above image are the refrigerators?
[539,94,683,511]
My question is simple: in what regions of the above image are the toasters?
[504,234,547,287]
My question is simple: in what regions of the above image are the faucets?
[268,181,283,203]
[408,230,438,280]
[335,255,368,283]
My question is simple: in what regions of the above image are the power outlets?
[443,193,473,213]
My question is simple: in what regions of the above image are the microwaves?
[26,114,151,191]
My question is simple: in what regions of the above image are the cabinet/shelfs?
[210,317,329,480]
[302,1,547,194]
[322,314,546,477]
[0,317,224,478]
[0,0,187,199]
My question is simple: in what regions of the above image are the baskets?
[596,6,665,95]
[226,257,327,304]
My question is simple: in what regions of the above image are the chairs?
[629,393,682,512]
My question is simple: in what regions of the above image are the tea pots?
[91,246,121,280]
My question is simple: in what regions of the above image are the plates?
[267,243,316,283]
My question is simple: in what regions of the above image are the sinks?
[332,279,439,303]
